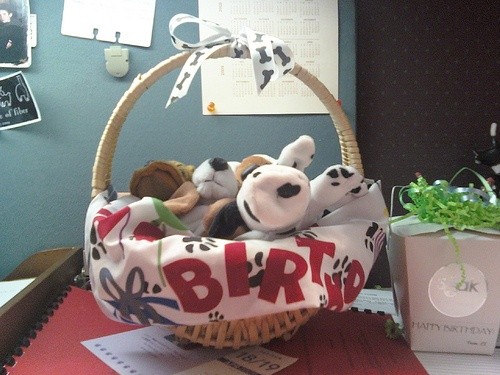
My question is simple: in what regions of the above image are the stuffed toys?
[130,134,373,241]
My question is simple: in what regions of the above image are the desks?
[0,245,500,375]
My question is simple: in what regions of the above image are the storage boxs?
[385,214,500,355]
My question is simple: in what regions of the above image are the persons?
[1,6,25,62]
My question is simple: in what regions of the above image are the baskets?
[89,38,371,350]
